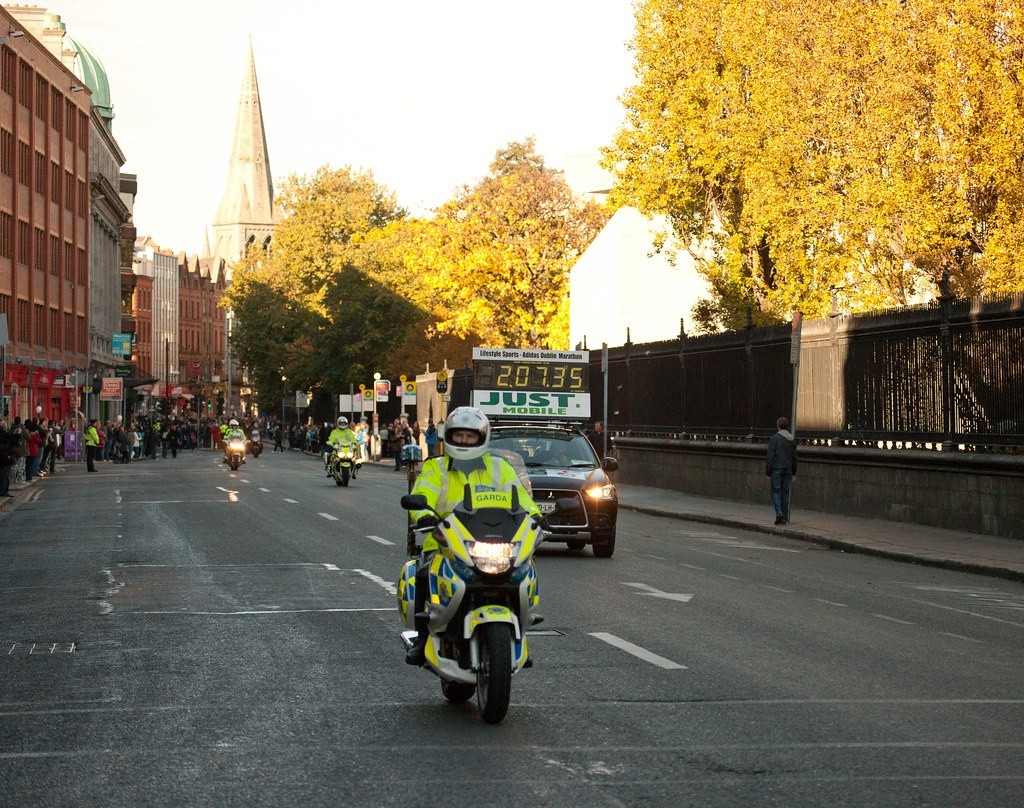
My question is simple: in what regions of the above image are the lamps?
[89,195,106,203]
[8,25,24,38]
[70,86,84,92]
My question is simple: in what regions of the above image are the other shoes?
[32,473,43,476]
[775,514,787,524]
[40,470,46,474]
[223,460,227,463]
[3,493,14,497]
[243,461,246,464]
[89,469,98,472]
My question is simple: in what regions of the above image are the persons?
[266,415,370,463]
[421,418,438,457]
[542,440,576,466]
[406,407,552,668]
[380,419,420,471]
[0,415,264,497]
[587,421,612,463]
[766,417,797,524]
[85,418,100,472]
[323,416,359,479]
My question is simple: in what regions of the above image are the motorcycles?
[321,440,364,487]
[245,431,265,458]
[397,480,581,724]
[221,432,251,470]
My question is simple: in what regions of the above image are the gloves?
[537,517,549,531]
[417,514,438,533]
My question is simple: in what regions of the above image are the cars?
[486,425,619,559]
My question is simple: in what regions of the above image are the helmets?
[229,419,239,429]
[337,416,348,429]
[444,406,491,460]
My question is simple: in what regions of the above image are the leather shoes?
[405,630,426,665]
[523,655,532,669]
